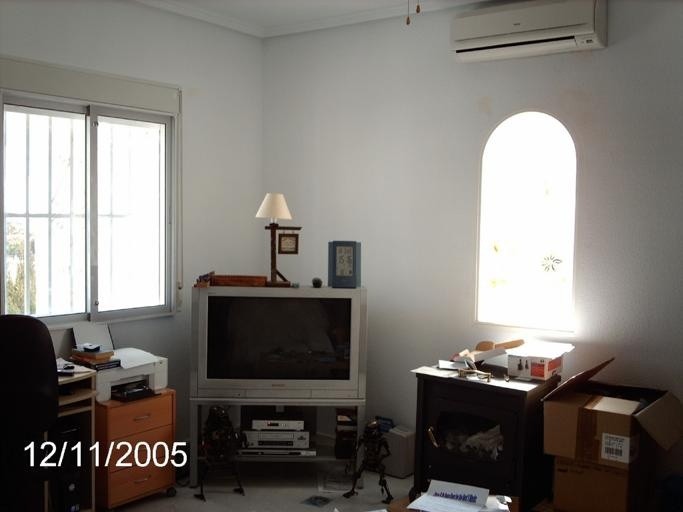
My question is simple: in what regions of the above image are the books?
[69,342,120,370]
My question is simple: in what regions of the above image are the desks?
[43,358,95,511]
[190,399,365,487]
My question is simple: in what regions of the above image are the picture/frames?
[278,233,298,254]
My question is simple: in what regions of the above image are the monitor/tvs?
[191,284,368,401]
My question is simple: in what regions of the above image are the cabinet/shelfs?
[416,380,556,512]
[96,388,176,509]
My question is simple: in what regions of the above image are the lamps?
[255,192,301,287]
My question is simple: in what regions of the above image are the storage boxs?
[541,357,683,511]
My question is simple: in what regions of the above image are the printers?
[66,321,169,403]
